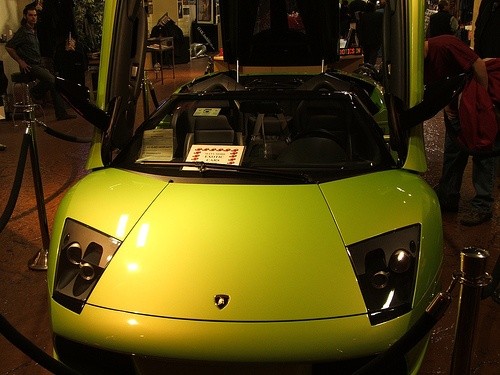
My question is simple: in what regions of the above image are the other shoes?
[56,111,76,120]
[28,90,46,104]
[438,195,458,213]
[461,211,493,226]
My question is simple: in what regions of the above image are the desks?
[145,37,175,85]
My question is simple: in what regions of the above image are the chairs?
[182,84,247,163]
[295,81,354,149]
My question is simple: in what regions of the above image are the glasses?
[445,4,450,6]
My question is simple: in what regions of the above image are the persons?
[426,0,459,39]
[6,5,76,120]
[432,0,500,224]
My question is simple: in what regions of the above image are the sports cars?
[45,60,447,371]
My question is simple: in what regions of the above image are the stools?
[10,71,46,127]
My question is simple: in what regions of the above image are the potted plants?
[73,0,106,65]
